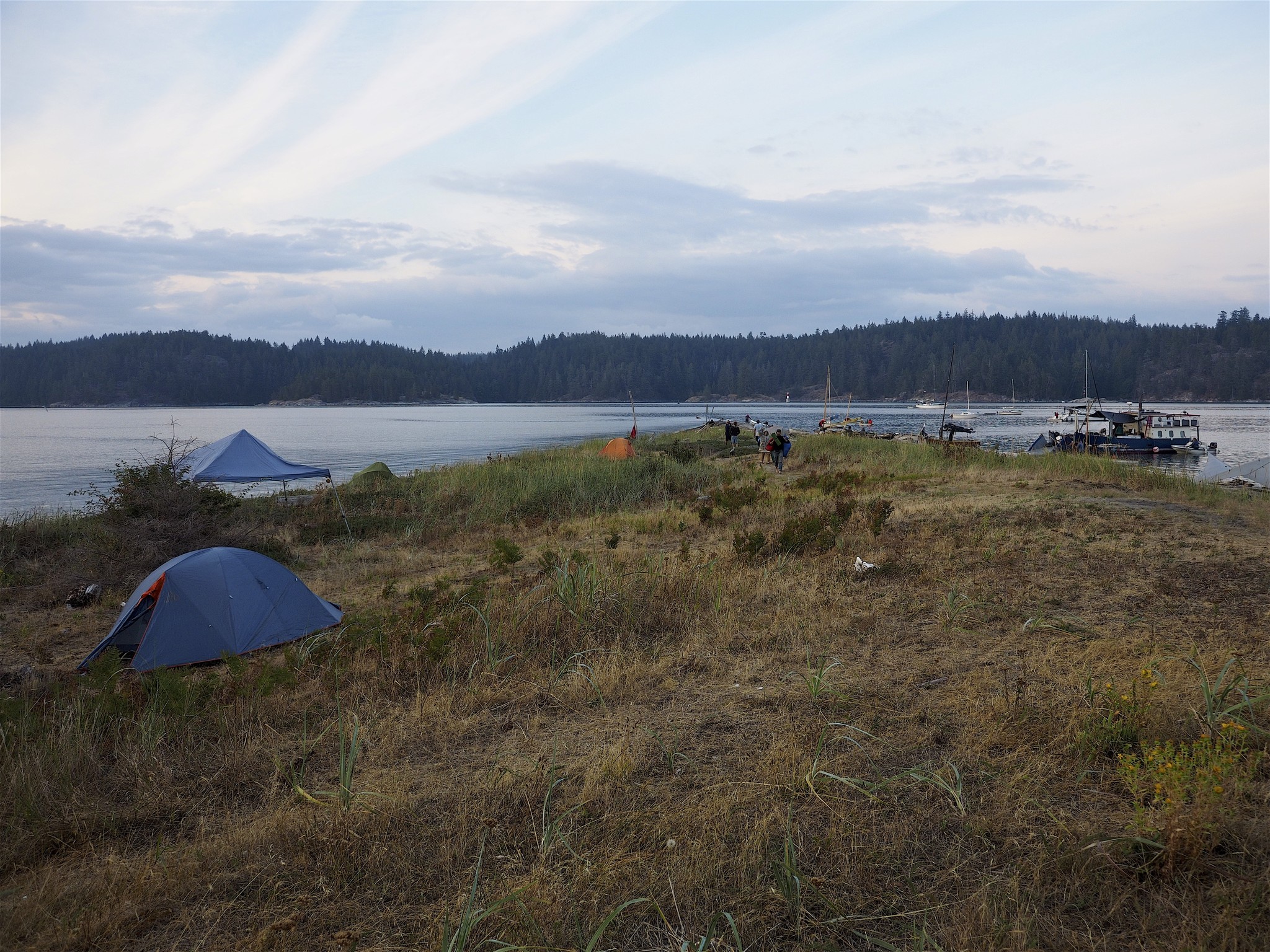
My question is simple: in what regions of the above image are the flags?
[630,423,637,439]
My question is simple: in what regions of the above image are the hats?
[727,419,731,423]
[776,429,781,434]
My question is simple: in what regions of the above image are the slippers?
[767,462,772,465]
[760,462,764,464]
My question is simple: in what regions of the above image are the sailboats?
[949,381,981,419]
[916,364,949,408]
[893,340,981,450]
[812,364,901,440]
[996,378,1024,415]
[1060,348,1109,422]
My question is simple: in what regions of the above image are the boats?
[1046,415,1064,422]
[750,420,769,429]
[1048,392,1201,454]
[906,406,915,408]
[788,428,811,437]
[1170,435,1220,455]
[1193,452,1270,497]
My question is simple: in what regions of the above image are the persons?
[1074,439,1083,453]
[698,420,714,429]
[725,414,789,474]
[1099,428,1106,435]
[1187,438,1199,449]
[844,426,867,436]
[1115,424,1123,436]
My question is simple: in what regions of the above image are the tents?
[596,437,636,460]
[172,429,353,541]
[75,547,345,675]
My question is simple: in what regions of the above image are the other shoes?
[779,470,782,474]
[776,468,779,473]
